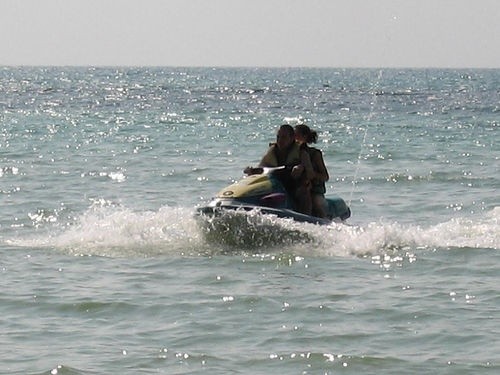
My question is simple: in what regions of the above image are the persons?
[243,124,316,215]
[292,124,330,218]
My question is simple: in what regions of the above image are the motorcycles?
[197,166,351,225]
